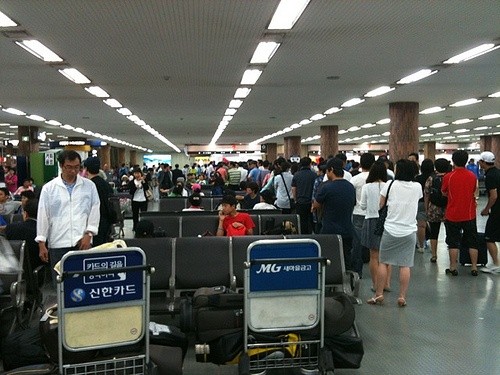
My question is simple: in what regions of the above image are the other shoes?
[480,193,486,196]
[430,255,437,263]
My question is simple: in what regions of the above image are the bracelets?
[218,227,223,230]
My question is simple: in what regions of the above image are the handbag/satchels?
[373,205,388,236]
[153,227,166,237]
[289,198,296,210]
[145,190,154,200]
[344,240,371,279]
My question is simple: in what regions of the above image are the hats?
[478,151,496,163]
[219,195,239,205]
[189,192,203,201]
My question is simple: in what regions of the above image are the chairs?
[0,184,365,375]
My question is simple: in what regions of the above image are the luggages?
[460,233,488,266]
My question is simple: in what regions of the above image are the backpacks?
[262,219,298,235]
[93,179,122,223]
[119,169,127,178]
[428,176,447,206]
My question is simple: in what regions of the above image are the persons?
[367,158,424,305]
[35,150,101,296]
[0,151,500,307]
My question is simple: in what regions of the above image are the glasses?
[62,165,80,171]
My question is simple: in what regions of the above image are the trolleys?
[39,247,158,375]
[239,237,332,374]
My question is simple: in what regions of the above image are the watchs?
[85,233,92,237]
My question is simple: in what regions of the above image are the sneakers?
[417,247,425,253]
[475,197,480,200]
[445,267,459,276]
[487,262,500,272]
[470,269,478,276]
